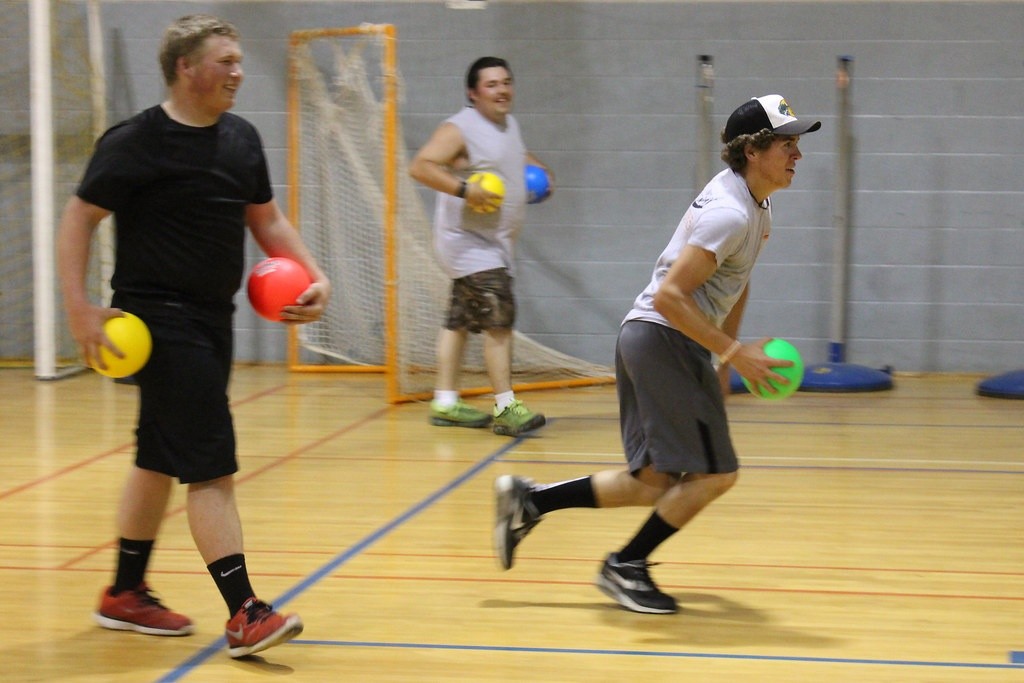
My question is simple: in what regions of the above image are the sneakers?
[492,397,545,434]
[596,555,676,613]
[225,597,304,660]
[427,400,490,427]
[496,477,540,570]
[95,582,194,635]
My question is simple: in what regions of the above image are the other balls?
[740,337,803,402]
[90,311,152,378]
[523,164,550,204]
[245,257,311,324]
[463,171,505,213]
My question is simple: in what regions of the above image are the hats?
[725,94,822,145]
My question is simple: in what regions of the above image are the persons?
[406,54,555,436]
[491,95,822,613]
[60,18,335,663]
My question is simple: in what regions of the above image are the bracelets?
[718,341,742,363]
[457,181,467,198]
